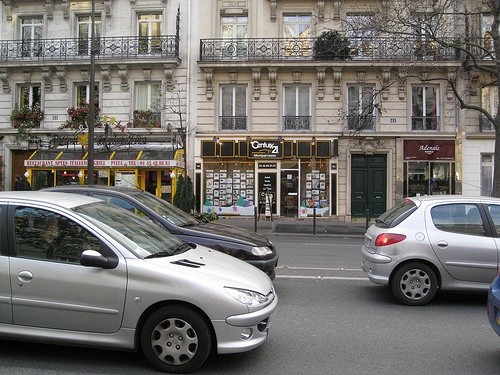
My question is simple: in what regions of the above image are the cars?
[359,193,500,305]
[0,191,277,375]
[34,185,279,290]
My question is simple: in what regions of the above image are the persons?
[14,174,31,191]
[23,217,60,260]
